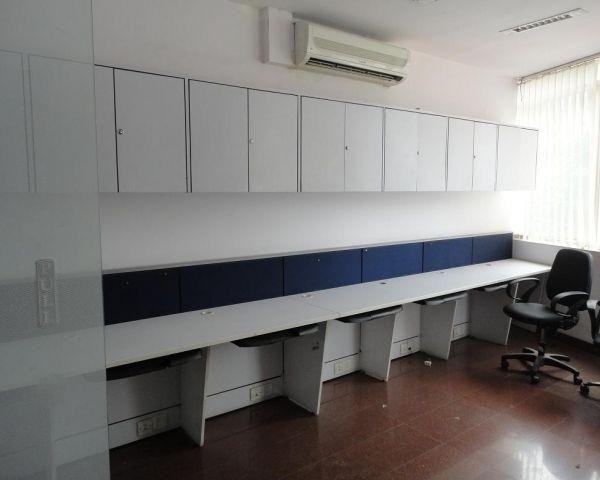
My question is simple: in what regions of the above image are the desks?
[1,257,561,478]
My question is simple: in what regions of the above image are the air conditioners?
[294,18,410,87]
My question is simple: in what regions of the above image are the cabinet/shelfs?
[448,116,540,194]
[383,105,447,195]
[300,96,383,194]
[6,50,188,194]
[189,78,300,194]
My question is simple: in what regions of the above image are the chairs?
[500,248,600,394]
